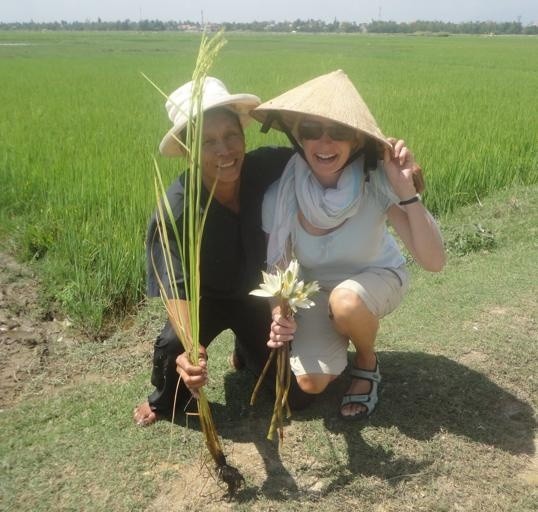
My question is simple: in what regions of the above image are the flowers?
[249,253,322,444]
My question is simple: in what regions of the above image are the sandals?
[340,352,383,419]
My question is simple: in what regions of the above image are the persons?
[248,68,447,420]
[132,73,425,427]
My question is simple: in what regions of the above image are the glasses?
[298,119,354,142]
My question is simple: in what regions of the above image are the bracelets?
[398,193,421,206]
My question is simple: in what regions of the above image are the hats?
[158,76,262,157]
[250,68,394,153]
